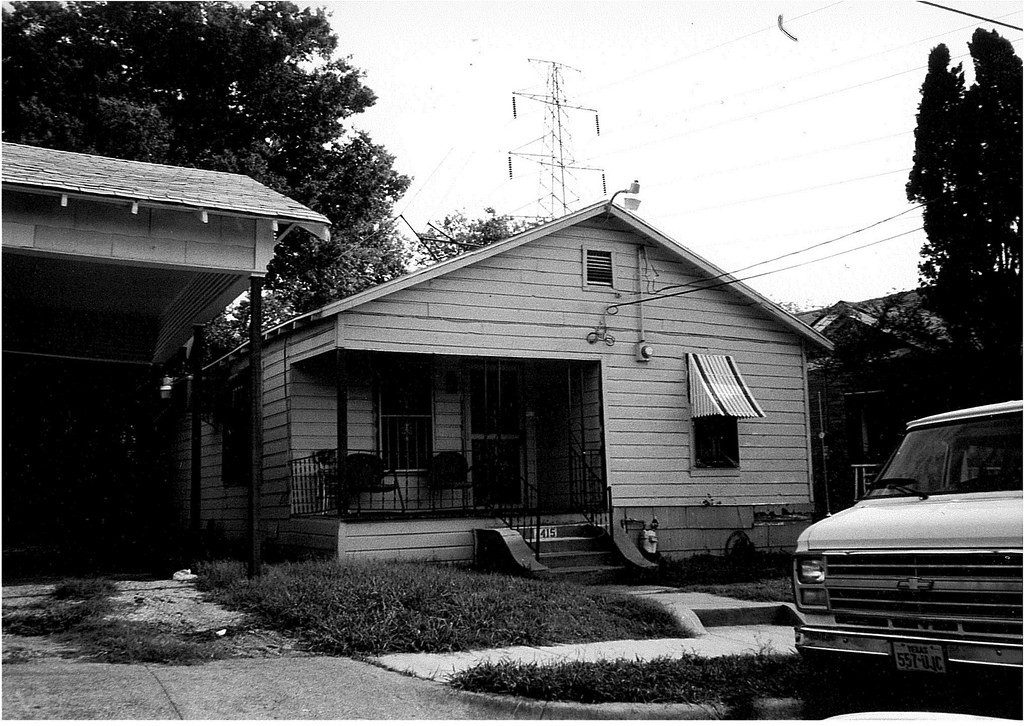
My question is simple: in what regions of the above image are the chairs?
[426,451,473,510]
[345,452,398,510]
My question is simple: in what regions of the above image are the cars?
[794,393,1022,686]
